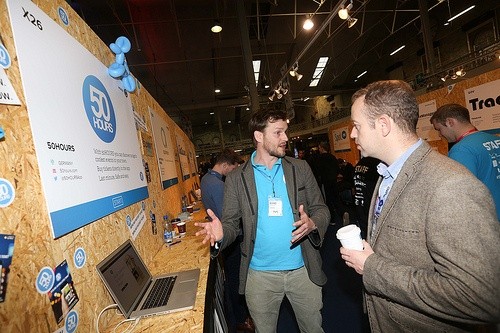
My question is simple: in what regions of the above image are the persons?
[200,149,255,333]
[348,156,389,240]
[429,103,500,222]
[194,104,344,333]
[340,79,500,333]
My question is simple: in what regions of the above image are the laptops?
[95,238,201,320]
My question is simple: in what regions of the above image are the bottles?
[181,196,188,212]
[163,215,173,243]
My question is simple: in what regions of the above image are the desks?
[100,197,210,333]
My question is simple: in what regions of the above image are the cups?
[170,218,181,232]
[187,205,194,215]
[177,222,187,236]
[336,224,363,251]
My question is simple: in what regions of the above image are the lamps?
[341,4,358,28]
[292,66,303,81]
[441,65,467,82]
[290,61,299,77]
[338,0,353,20]
[268,81,288,102]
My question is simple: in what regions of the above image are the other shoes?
[330,221,336,225]
